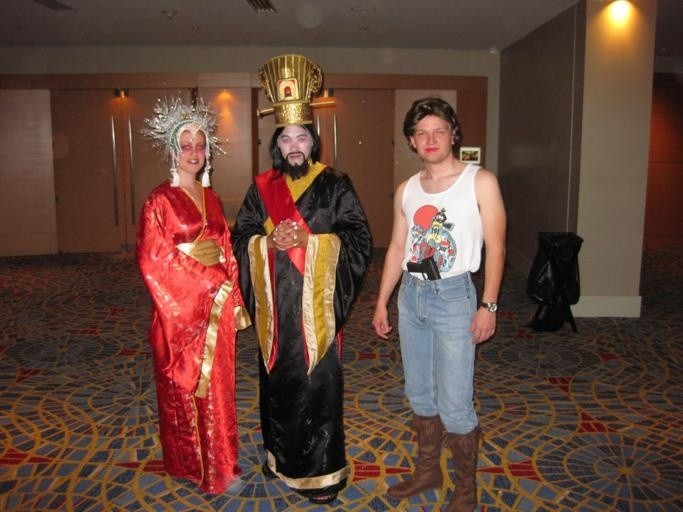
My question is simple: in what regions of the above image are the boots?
[388,416,478,512]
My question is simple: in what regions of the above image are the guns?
[407,257,441,281]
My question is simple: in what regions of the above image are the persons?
[372,97,508,512]
[231,125,372,504]
[137,127,252,493]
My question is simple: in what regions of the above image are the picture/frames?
[460,147,481,164]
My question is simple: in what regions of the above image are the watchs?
[480,302,499,312]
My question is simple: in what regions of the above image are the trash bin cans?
[527,231,584,333]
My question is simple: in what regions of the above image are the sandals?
[311,492,338,503]
